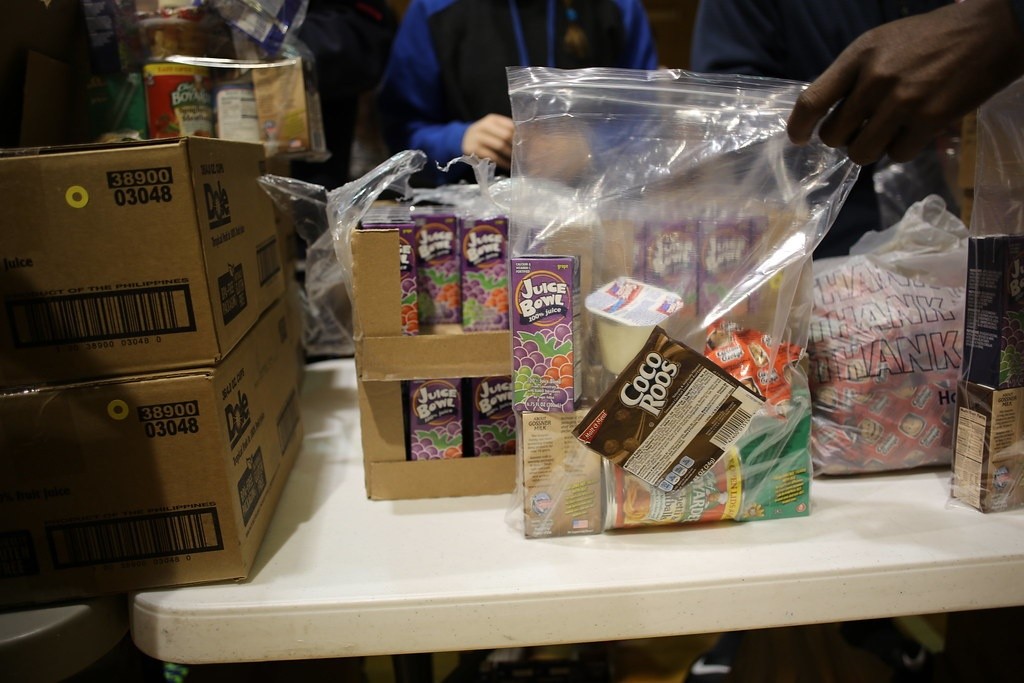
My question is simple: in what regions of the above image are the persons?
[374,0,659,193]
[284,0,399,191]
[690,0,1024,258]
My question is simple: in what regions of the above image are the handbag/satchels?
[812,193,975,477]
[949,81,1024,510]
[254,149,431,364]
[336,154,589,499]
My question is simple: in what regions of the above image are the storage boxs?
[1,136,302,608]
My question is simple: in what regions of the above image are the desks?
[131,354,1024,683]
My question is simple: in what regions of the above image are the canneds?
[142,61,263,143]
[600,445,746,529]
